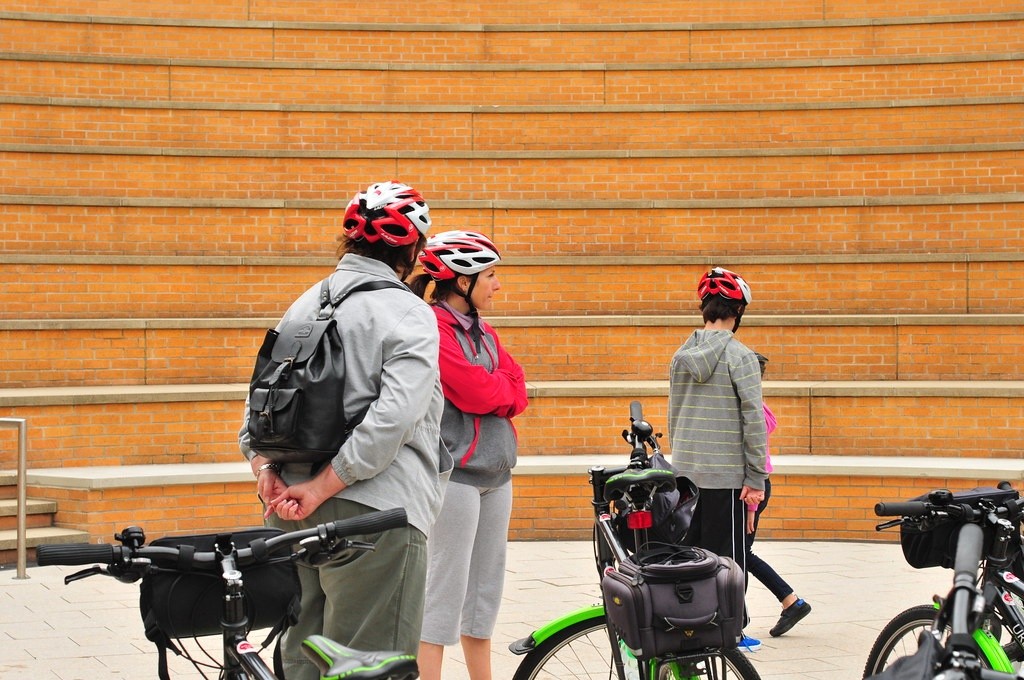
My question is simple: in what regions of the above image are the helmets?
[419,230,503,279]
[343,180,431,246]
[756,350,768,381]
[698,267,752,305]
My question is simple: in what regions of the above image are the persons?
[237,181,455,680]
[406,231,527,680]
[668,266,768,650]
[746,351,811,637]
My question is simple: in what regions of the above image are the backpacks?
[247,276,407,464]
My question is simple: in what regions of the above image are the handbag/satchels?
[602,542,747,660]
[900,485,1022,569]
[140,525,302,680]
[603,453,700,561]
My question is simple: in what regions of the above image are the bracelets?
[256,463,281,479]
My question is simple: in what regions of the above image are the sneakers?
[736,635,761,653]
[769,597,811,637]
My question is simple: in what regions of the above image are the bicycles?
[34,503,421,680]
[860,482,1024,680]
[510,401,760,680]
